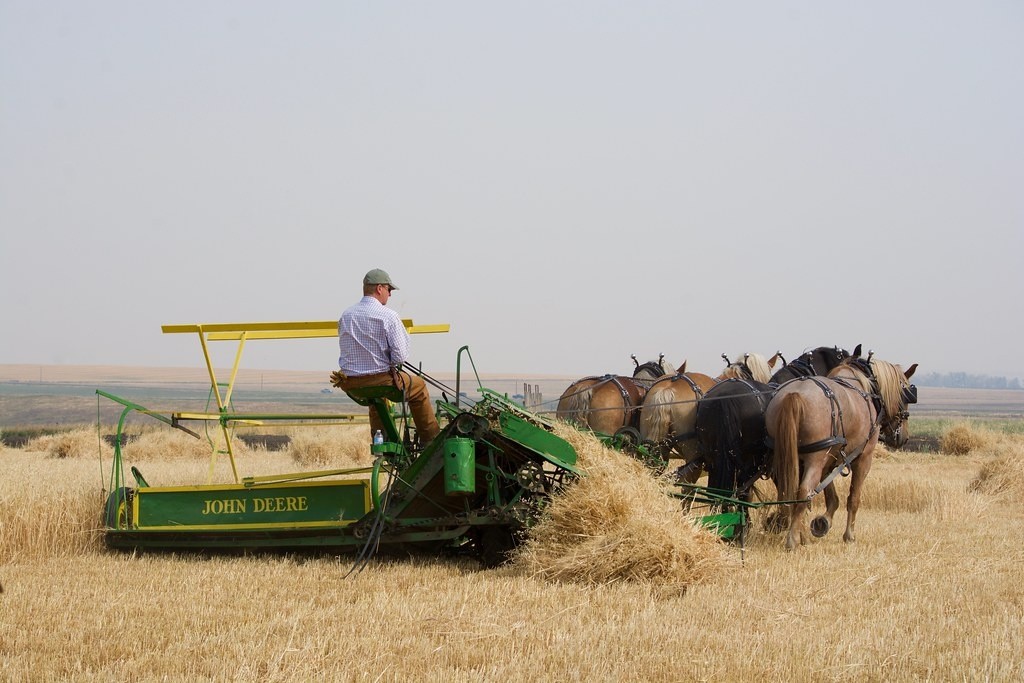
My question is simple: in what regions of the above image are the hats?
[362,268,399,290]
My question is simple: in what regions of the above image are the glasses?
[381,284,391,293]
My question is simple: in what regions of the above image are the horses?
[554,341,920,554]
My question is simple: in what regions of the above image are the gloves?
[330,371,347,388]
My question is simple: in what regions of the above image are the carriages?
[101,321,926,568]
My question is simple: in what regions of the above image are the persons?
[337,269,442,452]
